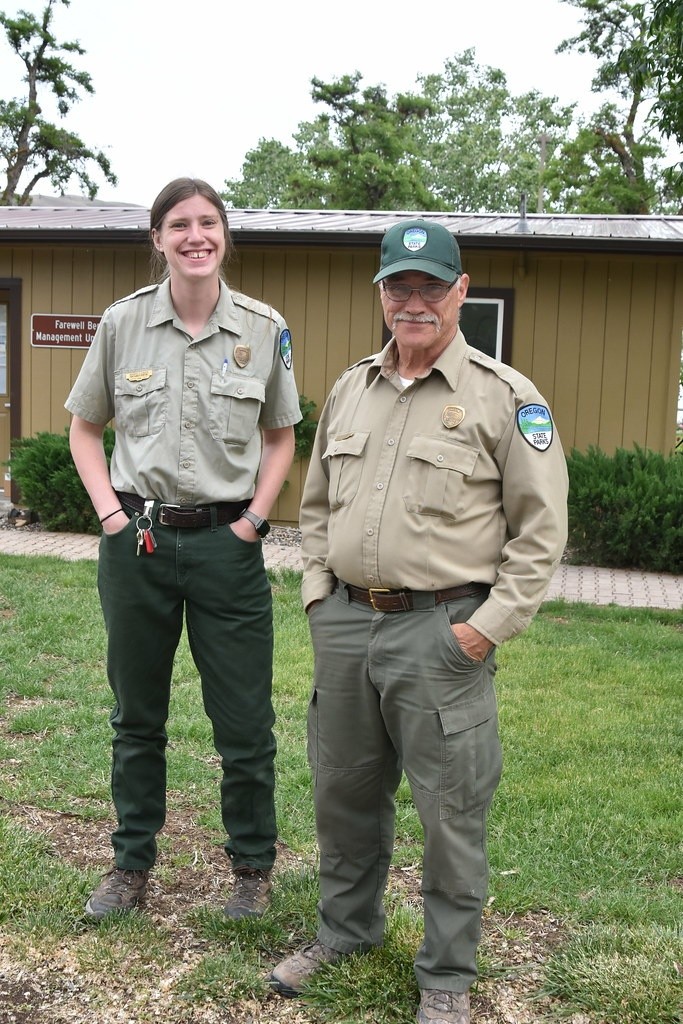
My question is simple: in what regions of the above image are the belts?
[334,578,490,612]
[114,491,253,528]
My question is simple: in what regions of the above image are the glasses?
[381,275,459,304]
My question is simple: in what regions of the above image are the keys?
[135,501,158,557]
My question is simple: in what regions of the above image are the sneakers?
[268,938,357,1000]
[86,866,151,920]
[415,986,471,1024]
[223,867,273,920]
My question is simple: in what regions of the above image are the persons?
[63,176,305,927]
[270,217,569,1024]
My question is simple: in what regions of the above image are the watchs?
[239,508,271,538]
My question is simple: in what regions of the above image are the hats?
[372,218,462,285]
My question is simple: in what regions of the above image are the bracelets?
[99,509,123,523]
